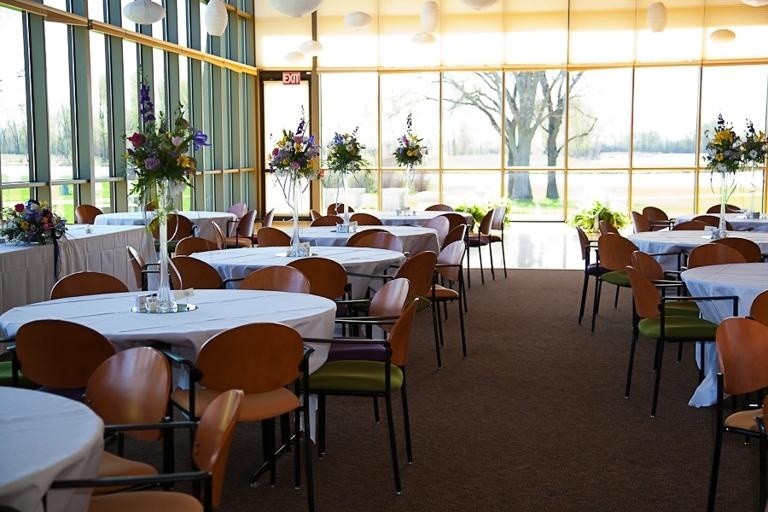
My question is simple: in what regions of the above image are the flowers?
[321,125,372,185]
[119,64,211,232]
[703,113,768,177]
[392,112,428,169]
[268,105,324,188]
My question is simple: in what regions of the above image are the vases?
[343,165,349,224]
[292,169,299,247]
[403,162,410,209]
[156,179,175,302]
[719,169,726,238]
[748,159,755,216]
